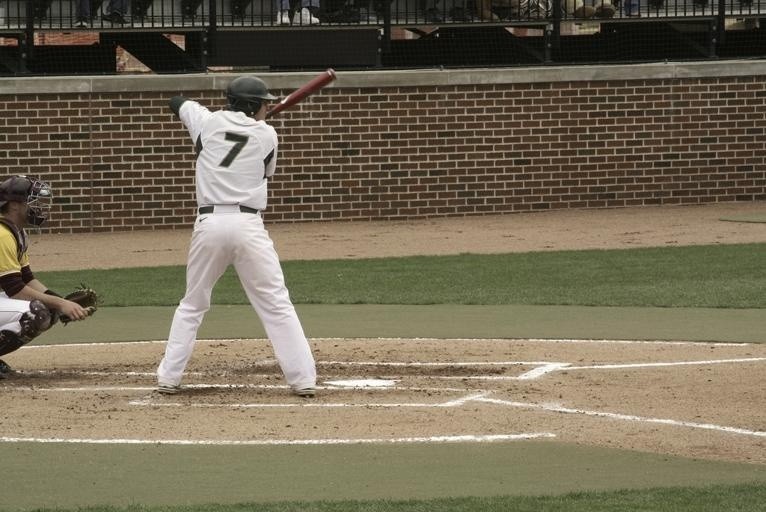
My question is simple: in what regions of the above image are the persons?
[156,76,317,396]
[0,175,97,380]
[25,0,620,26]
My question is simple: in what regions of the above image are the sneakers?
[276,8,291,26]
[231,8,247,20]
[573,5,597,18]
[73,15,88,27]
[100,12,148,24]
[291,384,317,396]
[593,6,616,18]
[182,6,197,19]
[292,8,320,26]
[33,7,47,21]
[0,358,14,380]
[156,380,181,395]
[423,6,502,21]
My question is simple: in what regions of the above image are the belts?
[197,204,262,216]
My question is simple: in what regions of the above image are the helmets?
[0,174,54,227]
[227,76,279,101]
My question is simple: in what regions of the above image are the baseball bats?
[265,68,337,120]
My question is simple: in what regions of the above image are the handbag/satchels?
[506,0,565,18]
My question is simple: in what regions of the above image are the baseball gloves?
[56,289,98,323]
